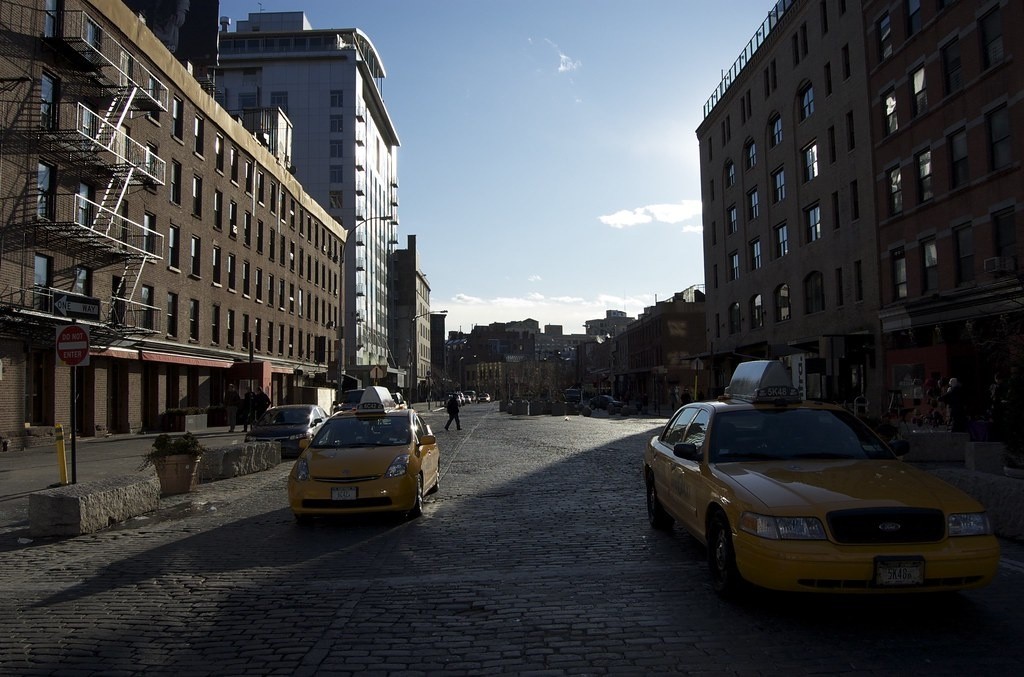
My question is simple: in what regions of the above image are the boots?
[251,424,253,431]
[241,423,248,432]
[229,426,235,432]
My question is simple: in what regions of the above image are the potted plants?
[163,407,208,431]
[139,431,209,494]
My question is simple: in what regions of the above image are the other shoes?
[457,428,462,430]
[444,427,449,431]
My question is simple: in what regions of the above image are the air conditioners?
[984,255,1015,273]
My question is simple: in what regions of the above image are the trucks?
[564,389,581,405]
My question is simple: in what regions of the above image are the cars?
[333,388,408,413]
[445,390,491,407]
[287,385,441,527]
[589,395,627,411]
[642,360,999,607]
[243,404,329,458]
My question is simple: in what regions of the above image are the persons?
[670,388,676,411]
[601,389,625,401]
[698,391,704,402]
[224,384,241,432]
[681,389,691,404]
[643,393,648,408]
[242,386,270,432]
[379,419,408,441]
[926,367,1024,442]
[444,393,462,430]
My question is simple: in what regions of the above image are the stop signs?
[55,325,90,366]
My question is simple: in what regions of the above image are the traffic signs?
[52,292,100,324]
